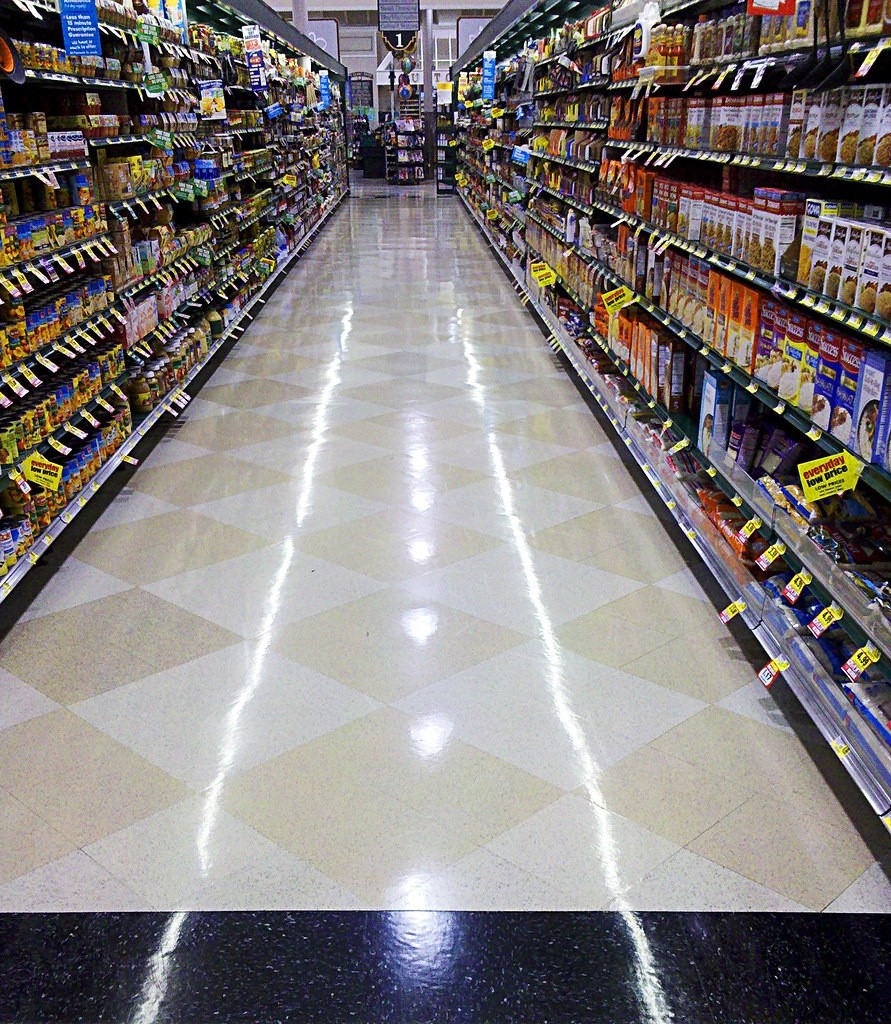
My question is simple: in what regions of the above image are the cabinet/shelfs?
[525,34,611,355]
[488,64,533,284]
[584,1,891,884]
[446,78,494,247]
[0,0,353,643]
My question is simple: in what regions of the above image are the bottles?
[647,24,685,84]
[129,309,224,412]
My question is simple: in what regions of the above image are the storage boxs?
[612,83,891,484]
